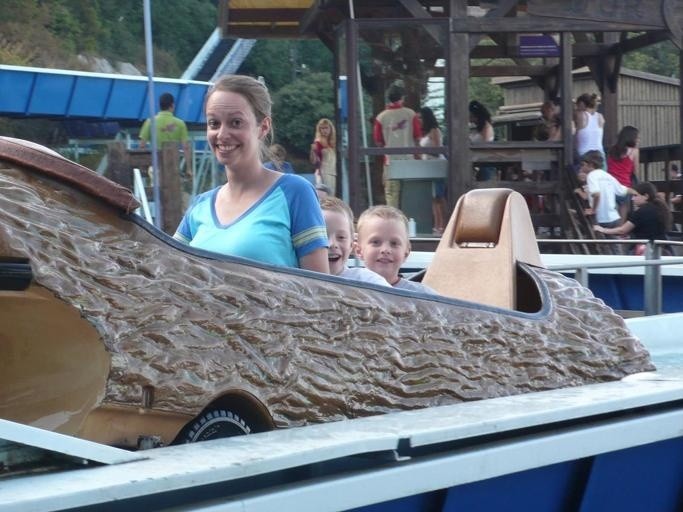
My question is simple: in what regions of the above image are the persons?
[263,121,441,295]
[169,74,331,276]
[138,92,194,187]
[516,88,682,258]
[375,82,498,234]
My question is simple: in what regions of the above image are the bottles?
[407,218,417,238]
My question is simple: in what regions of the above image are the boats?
[0,135,657,479]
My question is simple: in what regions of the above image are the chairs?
[419,188,543,314]
